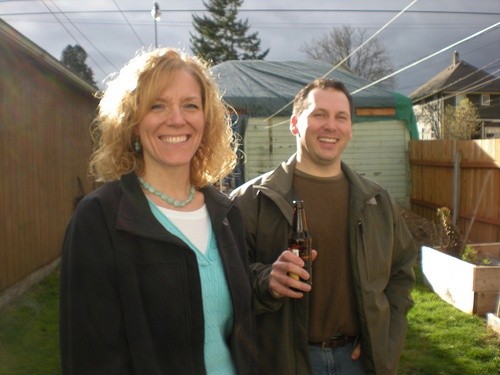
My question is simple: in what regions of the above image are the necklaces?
[137,176,196,207]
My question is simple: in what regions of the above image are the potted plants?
[420,206,500,317]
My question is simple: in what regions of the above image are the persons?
[227,78,419,375]
[57,46,261,375]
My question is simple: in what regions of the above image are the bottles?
[287,200,312,294]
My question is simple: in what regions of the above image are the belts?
[307,336,355,351]
